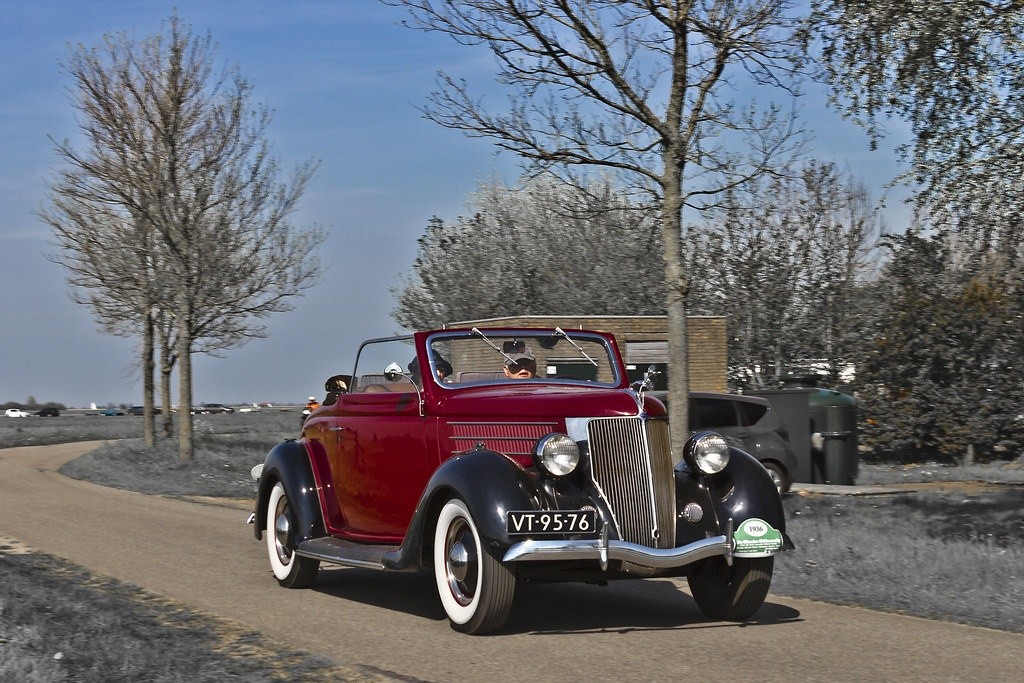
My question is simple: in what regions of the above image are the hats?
[408,341,453,377]
[504,344,535,365]
[309,396,316,401]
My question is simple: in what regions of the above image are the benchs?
[363,383,416,393]
[457,371,509,382]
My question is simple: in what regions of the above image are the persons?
[408,349,454,391]
[304,397,321,412]
[503,344,536,379]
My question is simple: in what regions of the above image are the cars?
[127,406,161,416]
[202,404,235,415]
[99,409,123,416]
[5,408,29,418]
[246,327,797,638]
[31,408,60,419]
